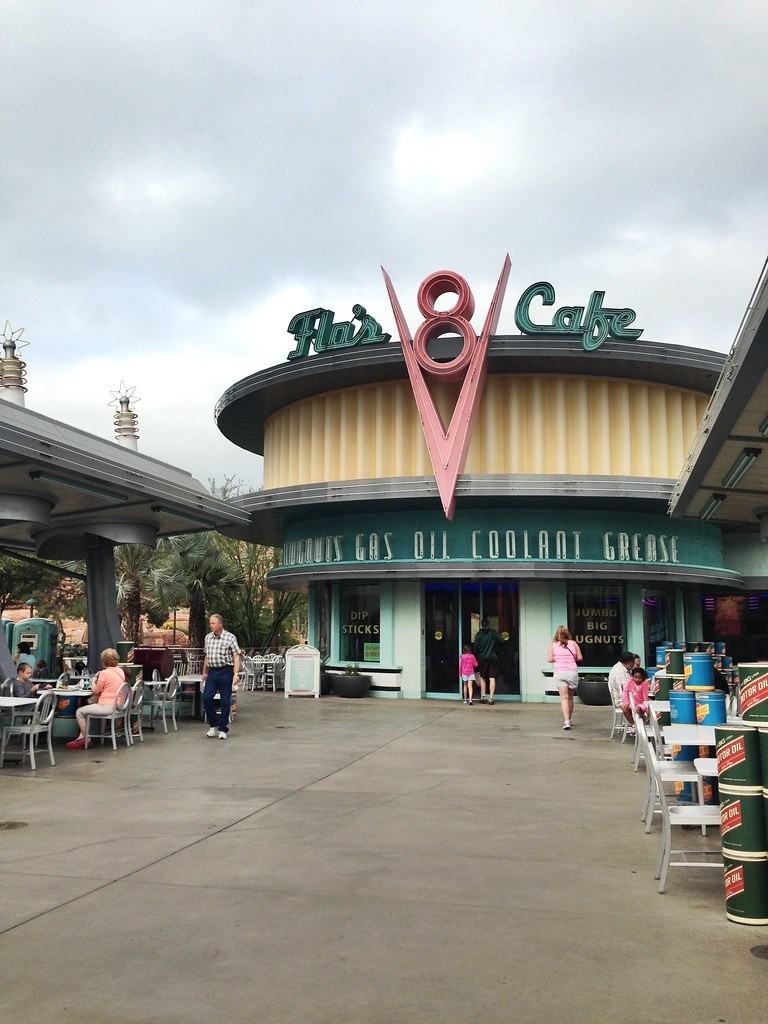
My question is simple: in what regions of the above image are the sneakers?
[207,726,218,737]
[219,731,227,739]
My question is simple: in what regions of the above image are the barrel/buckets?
[648,641,768,926]
[117,640,144,688]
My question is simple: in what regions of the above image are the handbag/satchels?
[87,694,100,703]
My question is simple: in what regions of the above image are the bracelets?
[234,673,239,676]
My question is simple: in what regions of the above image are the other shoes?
[88,738,93,748]
[463,700,467,703]
[570,719,572,725]
[66,738,85,748]
[563,722,570,730]
[630,727,636,737]
[489,699,494,704]
[469,699,472,705]
[480,697,487,702]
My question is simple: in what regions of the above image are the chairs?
[0,691,57,770]
[647,742,724,894]
[636,715,706,837]
[84,682,130,750]
[143,673,179,734]
[649,711,672,761]
[604,677,624,738]
[628,692,664,764]
[4,682,40,751]
[101,680,144,747]
[622,715,634,744]
[239,653,286,692]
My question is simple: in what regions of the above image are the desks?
[17,677,57,682]
[246,660,275,692]
[694,758,719,777]
[36,688,92,746]
[165,674,206,721]
[643,701,670,745]
[663,726,719,805]
[0,697,38,768]
[70,675,90,681]
[143,680,167,734]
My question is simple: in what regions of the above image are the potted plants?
[576,675,613,706]
[331,662,372,698]
[320,653,338,696]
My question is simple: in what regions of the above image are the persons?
[201,614,240,739]
[12,642,47,678]
[66,648,126,748]
[548,626,583,729]
[459,645,478,703]
[622,667,666,736]
[608,652,641,709]
[13,664,53,745]
[472,618,510,705]
